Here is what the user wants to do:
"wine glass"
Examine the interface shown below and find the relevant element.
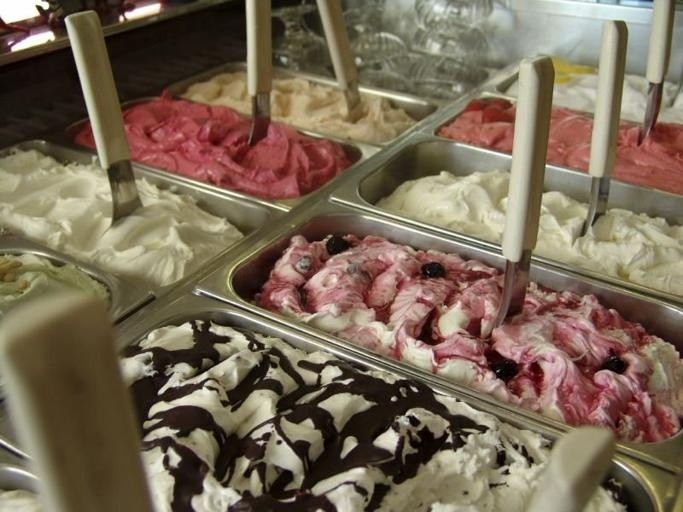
[270,1,496,102]
